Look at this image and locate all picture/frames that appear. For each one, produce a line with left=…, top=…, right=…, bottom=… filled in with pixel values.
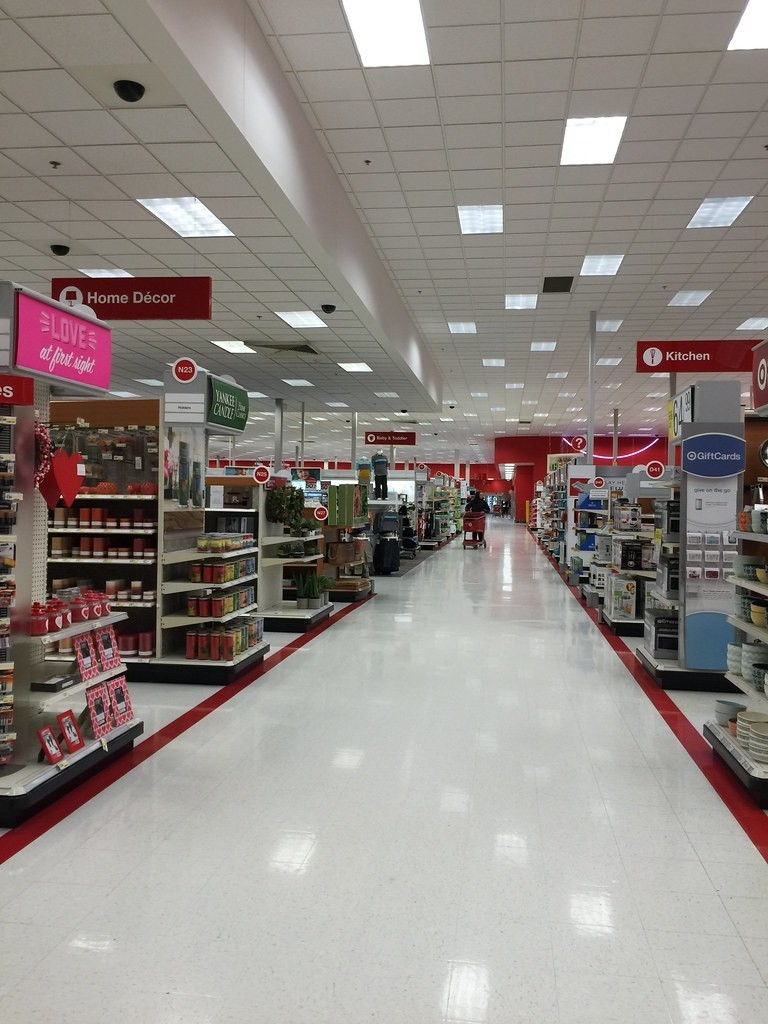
left=36, top=725, right=66, bottom=766
left=54, top=710, right=85, bottom=753
left=76, top=684, right=113, bottom=740
left=67, top=631, right=100, bottom=683
left=107, top=675, right=134, bottom=727
left=92, top=624, right=122, bottom=672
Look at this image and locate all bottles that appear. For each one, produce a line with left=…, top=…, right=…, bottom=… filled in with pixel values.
left=197, top=533, right=253, bottom=552
left=31, top=590, right=110, bottom=634
left=188, top=586, right=254, bottom=618
left=191, top=557, right=256, bottom=584
left=185, top=618, right=264, bottom=661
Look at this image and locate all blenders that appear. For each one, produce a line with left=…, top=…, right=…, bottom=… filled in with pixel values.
left=627, top=550, right=636, bottom=569
left=629, top=508, right=640, bottom=527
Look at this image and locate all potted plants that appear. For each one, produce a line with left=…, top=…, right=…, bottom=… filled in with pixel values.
left=304, top=545, right=315, bottom=556
left=314, top=575, right=328, bottom=606
left=301, top=518, right=314, bottom=538
left=315, top=521, right=322, bottom=535
left=309, top=571, right=321, bottom=609
left=314, top=545, right=321, bottom=555
left=320, top=577, right=335, bottom=604
left=292, top=572, right=309, bottom=608
left=277, top=544, right=291, bottom=558
left=291, top=543, right=305, bottom=558
left=280, top=487, right=306, bottom=537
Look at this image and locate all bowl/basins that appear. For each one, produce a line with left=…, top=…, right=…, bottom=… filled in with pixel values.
left=715, top=700, right=768, bottom=762
left=732, top=555, right=768, bottom=583
left=727, top=642, right=768, bottom=699
left=735, top=510, right=768, bottom=534
left=759, top=440, right=768, bottom=468
left=733, top=593, right=768, bottom=628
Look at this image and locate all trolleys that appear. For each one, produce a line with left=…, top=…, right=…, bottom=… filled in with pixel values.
left=492, top=505, right=501, bottom=518
left=463, top=512, right=486, bottom=550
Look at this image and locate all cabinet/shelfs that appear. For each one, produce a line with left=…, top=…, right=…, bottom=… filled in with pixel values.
left=528, top=338, right=768, bottom=812
left=0, top=281, right=477, bottom=829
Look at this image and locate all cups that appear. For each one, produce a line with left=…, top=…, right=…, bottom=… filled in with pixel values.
left=52, top=578, right=156, bottom=601
left=53, top=508, right=157, bottom=528
left=45, top=632, right=155, bottom=658
left=50, top=537, right=155, bottom=558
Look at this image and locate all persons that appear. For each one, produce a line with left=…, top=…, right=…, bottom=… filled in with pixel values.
left=464, top=491, right=490, bottom=549
left=371, top=449, right=390, bottom=500
left=296, top=469, right=316, bottom=488
left=234, top=468, right=249, bottom=475
left=354, top=457, right=373, bottom=500
left=501, top=499, right=511, bottom=520
